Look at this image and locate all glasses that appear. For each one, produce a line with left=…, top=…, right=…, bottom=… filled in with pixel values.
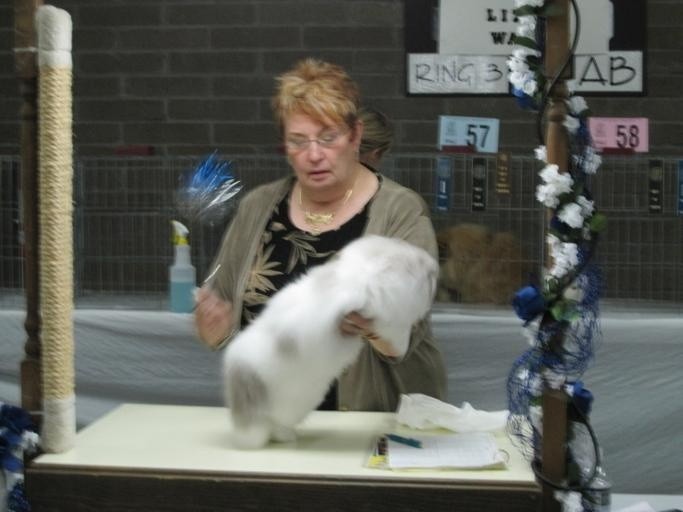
left=282, top=127, right=351, bottom=156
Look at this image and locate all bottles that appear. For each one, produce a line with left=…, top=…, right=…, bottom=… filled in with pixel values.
left=580, top=449, right=614, bottom=512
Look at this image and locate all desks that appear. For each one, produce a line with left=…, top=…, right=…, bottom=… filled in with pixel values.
left=26, top=403, right=546, bottom=512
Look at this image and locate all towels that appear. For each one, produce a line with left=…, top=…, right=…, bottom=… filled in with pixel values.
left=394, top=392, right=512, bottom=434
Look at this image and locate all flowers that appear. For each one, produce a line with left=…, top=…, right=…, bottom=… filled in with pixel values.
left=505, top=0, right=610, bottom=512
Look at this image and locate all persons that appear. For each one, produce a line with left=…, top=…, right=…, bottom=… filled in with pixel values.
left=193, top=58, right=447, bottom=410
left=354, top=107, right=400, bottom=169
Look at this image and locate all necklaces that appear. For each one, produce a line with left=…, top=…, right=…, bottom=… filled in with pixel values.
left=297, top=167, right=362, bottom=229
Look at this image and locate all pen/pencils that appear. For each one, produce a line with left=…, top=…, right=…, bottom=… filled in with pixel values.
left=385, top=435, right=421, bottom=448
